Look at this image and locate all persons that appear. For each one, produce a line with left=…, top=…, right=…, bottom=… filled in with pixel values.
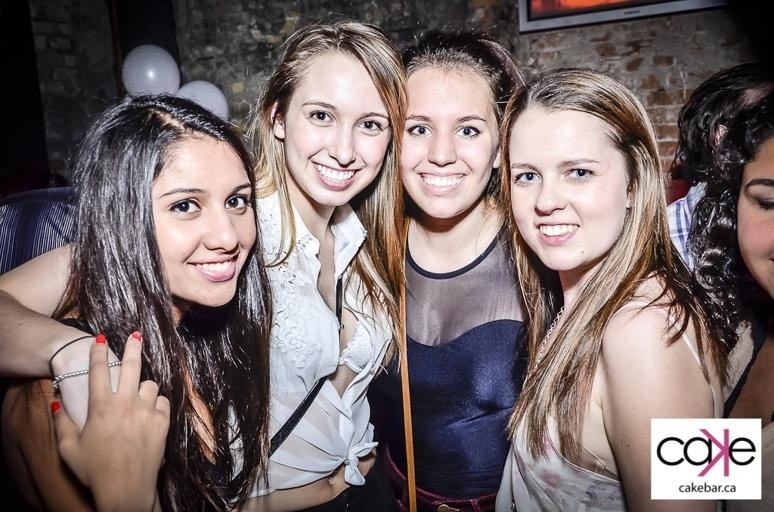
left=0, top=92, right=272, bottom=512
left=1, top=22, right=409, bottom=511
left=658, top=64, right=773, bottom=510
left=363, top=29, right=565, bottom=512
left=690, top=95, right=773, bottom=512
left=492, top=63, right=733, bottom=511
left=668, top=60, right=774, bottom=271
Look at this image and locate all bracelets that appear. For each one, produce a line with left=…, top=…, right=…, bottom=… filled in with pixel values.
left=48, top=358, right=121, bottom=389
left=48, top=334, right=94, bottom=361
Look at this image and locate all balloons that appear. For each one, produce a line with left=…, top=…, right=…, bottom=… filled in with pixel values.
left=121, top=43, right=180, bottom=99
left=178, top=80, right=231, bottom=124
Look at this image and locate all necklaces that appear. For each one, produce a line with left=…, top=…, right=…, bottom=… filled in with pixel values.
left=533, top=305, right=565, bottom=362
